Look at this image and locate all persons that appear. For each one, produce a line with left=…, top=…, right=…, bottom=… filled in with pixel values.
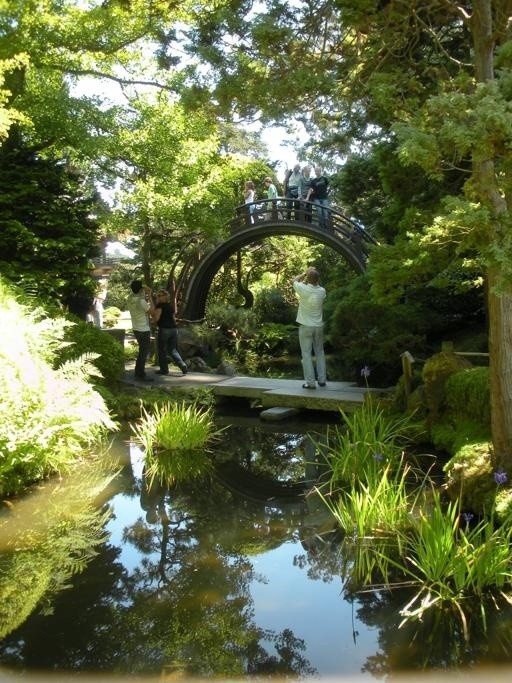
left=242, top=180, right=262, bottom=225
left=283, top=162, right=302, bottom=221
left=296, top=427, right=346, bottom=570
left=139, top=454, right=168, bottom=525
left=302, top=165, right=330, bottom=229
left=292, top=265, right=328, bottom=389
left=126, top=279, right=155, bottom=381
left=90, top=279, right=108, bottom=328
left=297, top=162, right=312, bottom=224
left=148, top=288, right=189, bottom=375
left=261, top=175, right=284, bottom=222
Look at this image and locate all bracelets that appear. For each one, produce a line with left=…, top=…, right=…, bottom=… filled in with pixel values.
left=303, top=271, right=306, bottom=276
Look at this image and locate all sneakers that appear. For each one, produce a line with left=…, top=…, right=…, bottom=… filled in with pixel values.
left=304, top=384, right=316, bottom=389
left=318, top=383, right=326, bottom=386
left=135, top=376, right=154, bottom=381
left=155, top=370, right=168, bottom=374
left=182, top=365, right=188, bottom=373
